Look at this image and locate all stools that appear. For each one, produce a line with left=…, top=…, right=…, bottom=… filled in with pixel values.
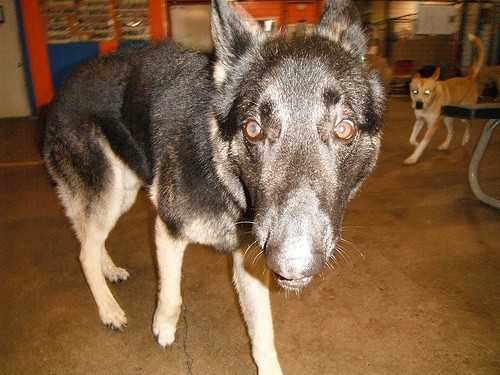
left=440, top=102, right=500, bottom=211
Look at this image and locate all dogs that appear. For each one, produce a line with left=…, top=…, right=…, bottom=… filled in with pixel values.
left=401, top=34, right=485, bottom=164
left=41, top=0, right=388, bottom=375
